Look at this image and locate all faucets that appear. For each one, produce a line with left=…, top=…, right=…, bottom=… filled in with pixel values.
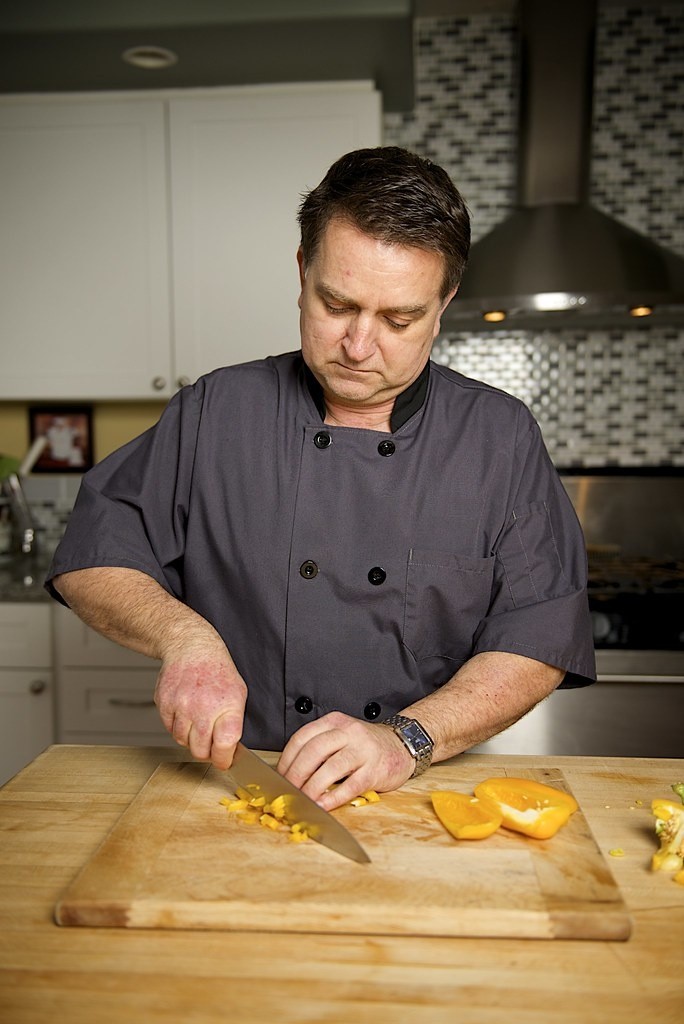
left=3, top=471, right=40, bottom=587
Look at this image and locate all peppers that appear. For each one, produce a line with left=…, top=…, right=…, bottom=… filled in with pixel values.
left=219, top=775, right=683, bottom=886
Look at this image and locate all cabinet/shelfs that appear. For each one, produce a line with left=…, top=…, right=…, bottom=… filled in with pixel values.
left=1, top=600, right=178, bottom=790
left=0, top=78, right=382, bottom=405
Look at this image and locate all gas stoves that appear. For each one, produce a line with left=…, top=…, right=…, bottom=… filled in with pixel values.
left=577, top=548, right=681, bottom=650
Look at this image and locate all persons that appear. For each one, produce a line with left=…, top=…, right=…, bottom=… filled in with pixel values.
left=37, top=143, right=596, bottom=817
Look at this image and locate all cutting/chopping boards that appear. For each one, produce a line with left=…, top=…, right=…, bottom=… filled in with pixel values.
left=55, top=753, right=630, bottom=942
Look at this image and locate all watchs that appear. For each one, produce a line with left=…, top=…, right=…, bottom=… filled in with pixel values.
left=380, top=716, right=435, bottom=779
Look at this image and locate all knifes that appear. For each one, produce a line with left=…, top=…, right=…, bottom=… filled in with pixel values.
left=223, top=744, right=371, bottom=867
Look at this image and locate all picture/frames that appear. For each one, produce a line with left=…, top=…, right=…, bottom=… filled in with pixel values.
left=25, top=400, right=96, bottom=476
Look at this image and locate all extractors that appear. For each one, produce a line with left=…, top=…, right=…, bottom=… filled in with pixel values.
left=421, top=0, right=684, bottom=332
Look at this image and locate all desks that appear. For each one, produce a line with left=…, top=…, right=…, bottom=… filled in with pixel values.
left=0, top=742, right=684, bottom=1024
